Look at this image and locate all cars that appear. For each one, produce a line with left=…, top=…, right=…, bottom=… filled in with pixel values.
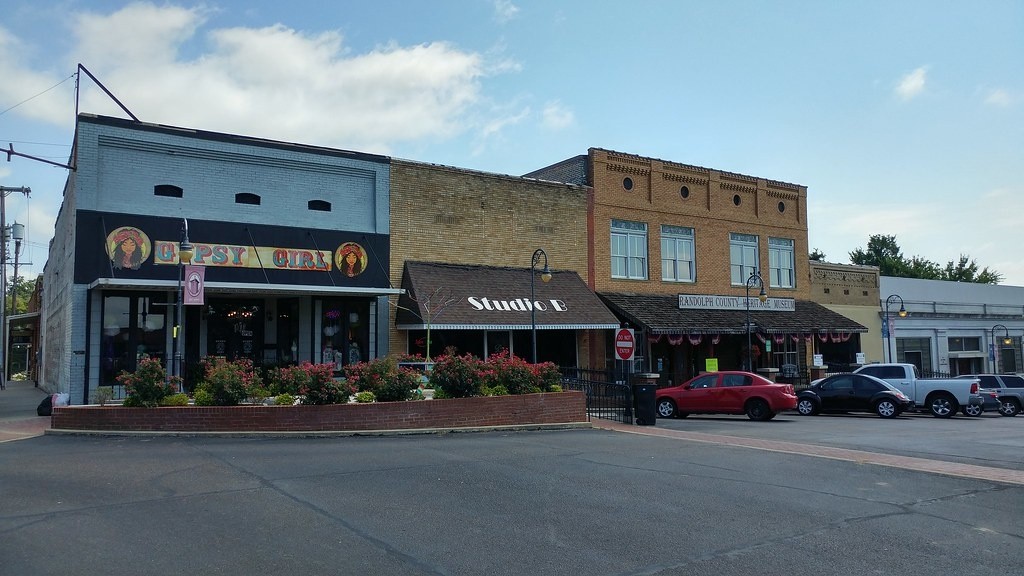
left=962, top=388, right=1002, bottom=417
left=952, top=372, right=1024, bottom=417
left=656, top=371, right=798, bottom=421
left=103, top=345, right=165, bottom=375
left=795, top=374, right=915, bottom=419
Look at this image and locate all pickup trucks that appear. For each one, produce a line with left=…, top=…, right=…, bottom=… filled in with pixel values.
left=811, top=362, right=984, bottom=419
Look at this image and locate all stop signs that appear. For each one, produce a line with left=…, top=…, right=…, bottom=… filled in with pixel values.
left=615, top=328, right=635, bottom=361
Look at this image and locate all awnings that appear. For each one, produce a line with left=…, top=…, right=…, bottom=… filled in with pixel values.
left=595, top=291, right=869, bottom=333
left=89, top=216, right=406, bottom=296
left=395, top=261, right=620, bottom=330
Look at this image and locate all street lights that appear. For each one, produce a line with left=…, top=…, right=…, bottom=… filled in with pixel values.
left=886, top=294, right=907, bottom=362
left=173, top=217, right=194, bottom=393
left=531, top=249, right=552, bottom=363
left=992, top=325, right=1012, bottom=373
left=747, top=274, right=767, bottom=371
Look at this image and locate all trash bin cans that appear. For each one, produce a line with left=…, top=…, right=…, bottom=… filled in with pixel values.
left=632, top=384, right=657, bottom=425
